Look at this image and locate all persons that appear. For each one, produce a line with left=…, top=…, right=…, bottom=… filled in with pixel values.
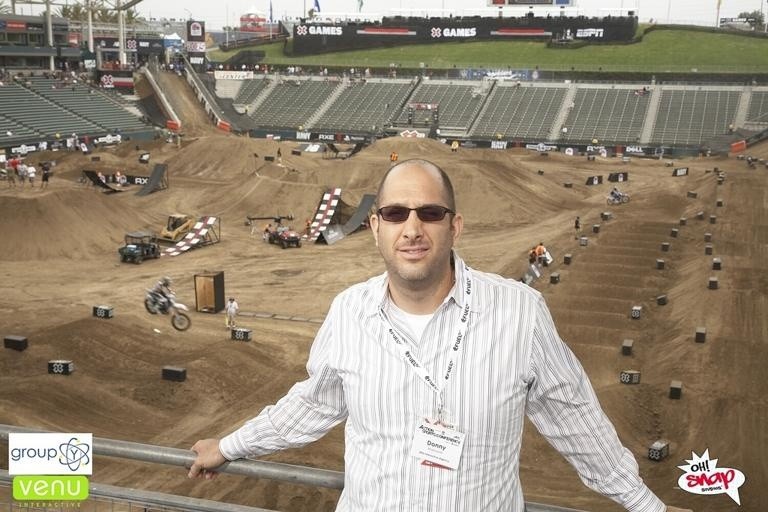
left=152, top=276, right=175, bottom=315
left=536, top=243, right=546, bottom=267
left=528, top=247, right=537, bottom=266
left=263, top=224, right=272, bottom=240
left=186, top=159, right=695, bottom=512
left=390, top=151, right=398, bottom=166
left=1, top=69, right=121, bottom=190
left=305, top=218, right=312, bottom=239
left=574, top=216, right=581, bottom=240
left=611, top=187, right=620, bottom=202
left=225, top=297, right=239, bottom=328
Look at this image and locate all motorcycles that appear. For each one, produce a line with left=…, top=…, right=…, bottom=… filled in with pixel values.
left=143, top=287, right=191, bottom=331
left=606, top=192, right=630, bottom=206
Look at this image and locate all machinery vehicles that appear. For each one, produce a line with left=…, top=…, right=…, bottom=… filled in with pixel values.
left=149, top=213, right=196, bottom=248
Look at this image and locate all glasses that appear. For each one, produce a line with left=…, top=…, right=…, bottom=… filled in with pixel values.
left=377, top=205, right=455, bottom=221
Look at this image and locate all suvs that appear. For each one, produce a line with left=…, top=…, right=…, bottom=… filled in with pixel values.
left=269, top=225, right=301, bottom=248
left=118, top=231, right=160, bottom=264
